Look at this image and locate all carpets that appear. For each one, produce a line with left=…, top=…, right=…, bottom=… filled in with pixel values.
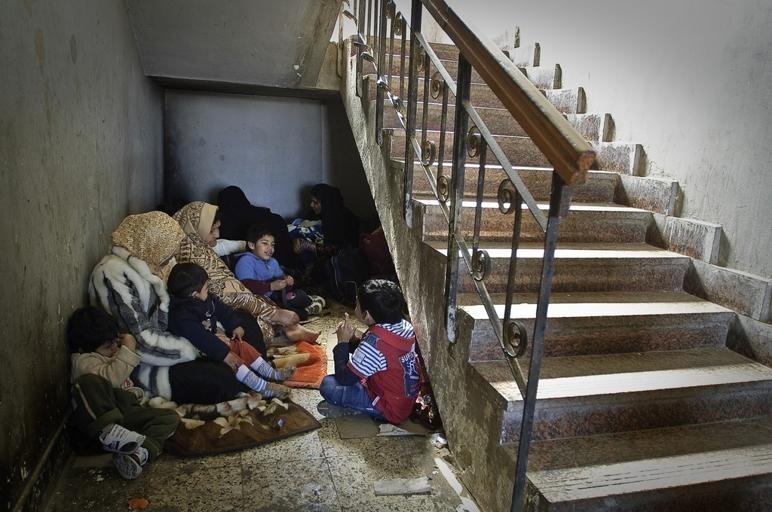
left=279, top=342, right=327, bottom=387
left=161, top=396, right=320, bottom=458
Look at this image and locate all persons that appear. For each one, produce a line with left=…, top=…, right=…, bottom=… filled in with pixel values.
left=88, top=186, right=326, bottom=400
left=61, top=306, right=179, bottom=480
left=290, top=184, right=361, bottom=299
left=319, top=278, right=422, bottom=425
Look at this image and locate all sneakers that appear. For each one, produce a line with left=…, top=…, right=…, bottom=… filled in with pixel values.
left=269, top=367, right=295, bottom=382
left=113, top=447, right=149, bottom=480
left=265, top=383, right=291, bottom=399
left=99, top=425, right=146, bottom=456
left=306, top=294, right=326, bottom=315
